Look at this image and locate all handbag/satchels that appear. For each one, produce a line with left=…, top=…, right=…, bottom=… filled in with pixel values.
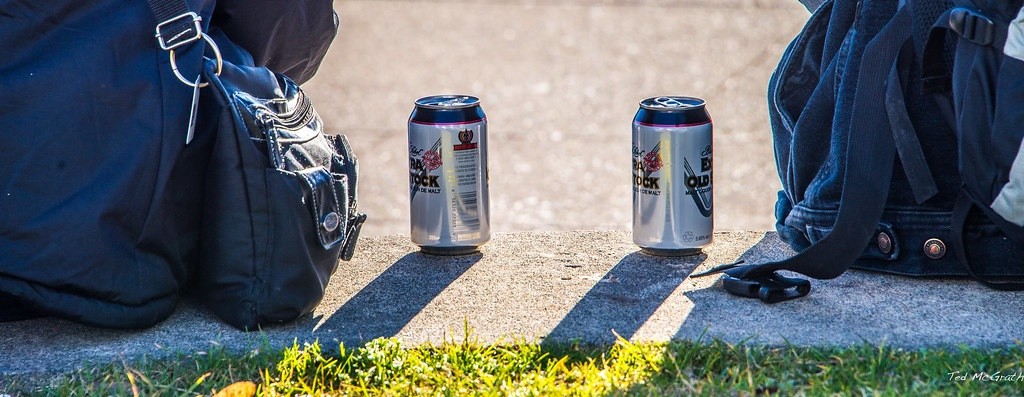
left=200, top=20, right=368, bottom=332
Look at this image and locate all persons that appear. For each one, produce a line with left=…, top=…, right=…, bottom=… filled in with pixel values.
left=769, top=0, right=1024, bottom=285
left=0, top=0, right=338, bottom=329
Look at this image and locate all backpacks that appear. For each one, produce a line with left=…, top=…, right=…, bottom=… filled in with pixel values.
left=722, top=0, right=1023, bottom=305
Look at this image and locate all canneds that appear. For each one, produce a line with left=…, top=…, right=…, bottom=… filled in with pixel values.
left=632, top=96, right=713, bottom=256
left=407, top=95, right=489, bottom=254
